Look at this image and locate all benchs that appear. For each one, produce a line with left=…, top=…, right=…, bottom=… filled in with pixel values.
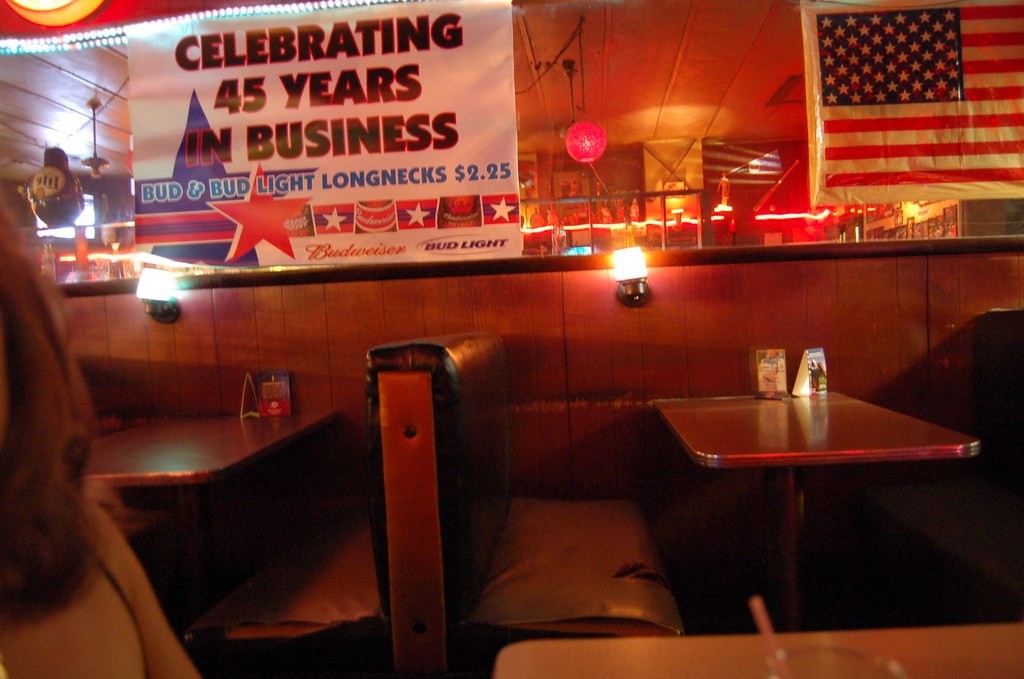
left=76, top=292, right=1024, bottom=677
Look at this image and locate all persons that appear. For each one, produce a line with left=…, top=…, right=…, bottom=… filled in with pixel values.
left=0, top=250, right=204, bottom=679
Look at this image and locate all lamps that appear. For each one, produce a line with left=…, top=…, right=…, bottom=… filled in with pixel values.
left=610, top=244, right=655, bottom=306
left=137, top=266, right=184, bottom=323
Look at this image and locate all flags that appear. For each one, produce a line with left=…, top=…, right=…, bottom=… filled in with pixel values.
left=799, top=0, right=1024, bottom=206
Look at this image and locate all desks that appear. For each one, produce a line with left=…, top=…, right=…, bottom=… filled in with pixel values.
left=657, top=392, right=985, bottom=627
left=81, top=403, right=345, bottom=619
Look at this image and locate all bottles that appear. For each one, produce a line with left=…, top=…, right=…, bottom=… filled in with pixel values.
left=42, top=243, right=57, bottom=281
